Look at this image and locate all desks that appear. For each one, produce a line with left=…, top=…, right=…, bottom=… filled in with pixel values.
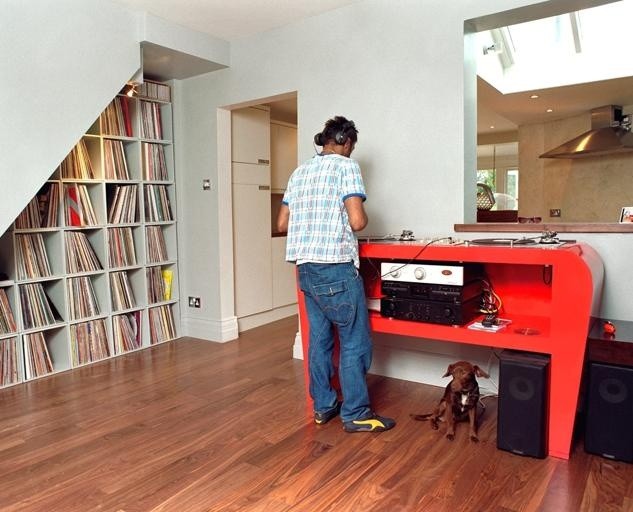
left=295, top=240, right=605, bottom=460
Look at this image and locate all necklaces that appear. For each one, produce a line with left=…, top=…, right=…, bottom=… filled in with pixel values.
left=321, top=148, right=336, bottom=154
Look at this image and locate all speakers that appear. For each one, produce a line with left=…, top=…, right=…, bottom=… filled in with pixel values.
left=496, top=348, right=550, bottom=460
left=584, top=362, right=633, bottom=463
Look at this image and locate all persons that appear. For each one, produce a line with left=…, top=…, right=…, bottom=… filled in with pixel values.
left=276, top=114, right=396, bottom=434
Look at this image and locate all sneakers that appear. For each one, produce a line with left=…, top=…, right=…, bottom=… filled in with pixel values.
left=342, top=415, right=396, bottom=434
left=313, top=400, right=341, bottom=425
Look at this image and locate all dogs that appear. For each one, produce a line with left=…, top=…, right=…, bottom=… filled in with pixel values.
left=408, top=360, right=491, bottom=443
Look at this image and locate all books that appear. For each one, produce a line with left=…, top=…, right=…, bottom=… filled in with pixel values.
left=100, top=81, right=178, bottom=355
left=0, top=280, right=18, bottom=385
left=15, top=183, right=99, bottom=229
left=61, top=138, right=95, bottom=179
left=17, top=231, right=101, bottom=382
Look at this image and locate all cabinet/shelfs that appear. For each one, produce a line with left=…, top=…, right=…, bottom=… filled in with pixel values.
left=1, top=80, right=181, bottom=390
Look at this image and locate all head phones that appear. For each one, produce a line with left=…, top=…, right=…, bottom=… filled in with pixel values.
left=313, top=120, right=355, bottom=145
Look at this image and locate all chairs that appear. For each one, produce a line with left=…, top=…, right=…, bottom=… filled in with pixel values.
left=231, top=104, right=298, bottom=333
